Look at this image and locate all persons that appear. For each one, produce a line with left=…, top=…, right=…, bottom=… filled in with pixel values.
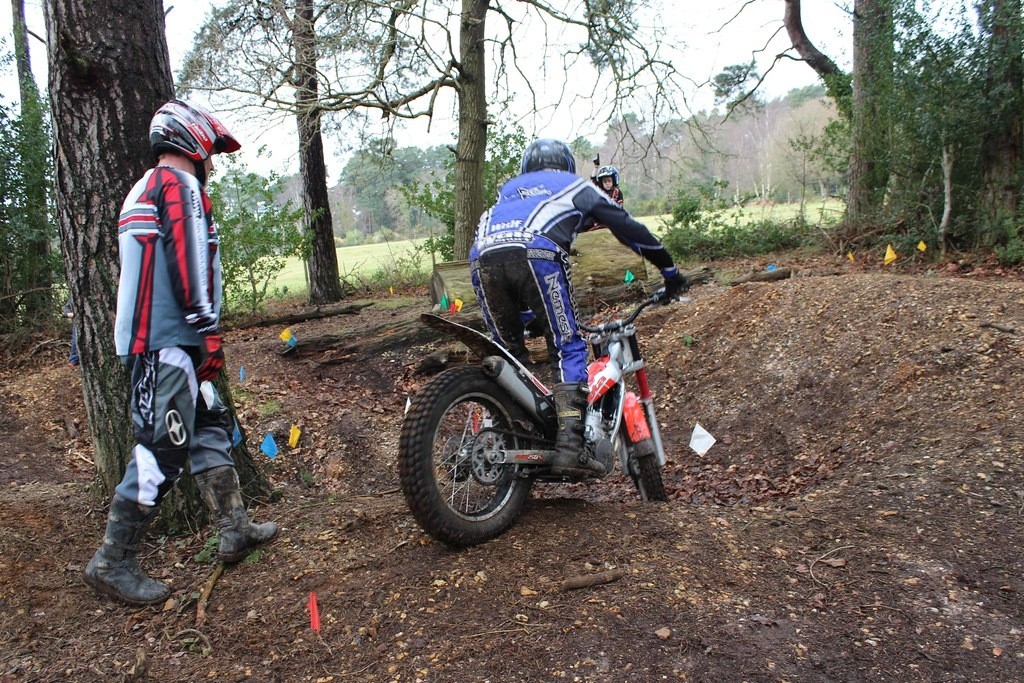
left=587, top=153, right=623, bottom=231
left=65, top=298, right=80, bottom=367
left=82, top=98, right=282, bottom=605
left=469, top=139, right=686, bottom=479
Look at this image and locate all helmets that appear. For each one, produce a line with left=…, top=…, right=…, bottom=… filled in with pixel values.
left=596, top=166, right=619, bottom=189
left=149, top=99, right=241, bottom=161
left=521, top=138, right=576, bottom=174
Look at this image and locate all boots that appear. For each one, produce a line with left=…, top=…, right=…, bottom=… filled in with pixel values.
left=82, top=493, right=170, bottom=606
left=552, top=381, right=605, bottom=478
left=195, top=465, right=280, bottom=562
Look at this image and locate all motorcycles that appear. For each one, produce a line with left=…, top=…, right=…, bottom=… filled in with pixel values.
left=393, top=278, right=694, bottom=547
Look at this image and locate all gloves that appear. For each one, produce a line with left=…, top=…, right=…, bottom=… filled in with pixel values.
left=663, top=271, right=690, bottom=307
left=525, top=317, right=545, bottom=339
left=593, top=153, right=599, bottom=168
left=195, top=329, right=225, bottom=385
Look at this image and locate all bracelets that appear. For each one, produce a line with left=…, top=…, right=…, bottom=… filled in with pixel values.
left=196, top=324, right=218, bottom=335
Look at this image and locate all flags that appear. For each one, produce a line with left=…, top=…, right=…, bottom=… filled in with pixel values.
left=280, top=327, right=297, bottom=348
left=849, top=251, right=855, bottom=267
left=766, top=263, right=778, bottom=271
left=624, top=269, right=635, bottom=285
left=441, top=286, right=463, bottom=315
left=306, top=591, right=320, bottom=635
left=289, top=424, right=301, bottom=449
left=884, top=243, right=897, bottom=266
left=239, top=365, right=245, bottom=383
left=390, top=286, right=393, bottom=296
left=260, top=432, right=278, bottom=459
left=690, top=424, right=716, bottom=458
left=917, top=240, right=927, bottom=251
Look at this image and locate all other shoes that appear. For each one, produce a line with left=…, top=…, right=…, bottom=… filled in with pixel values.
left=67, top=362, right=80, bottom=367
